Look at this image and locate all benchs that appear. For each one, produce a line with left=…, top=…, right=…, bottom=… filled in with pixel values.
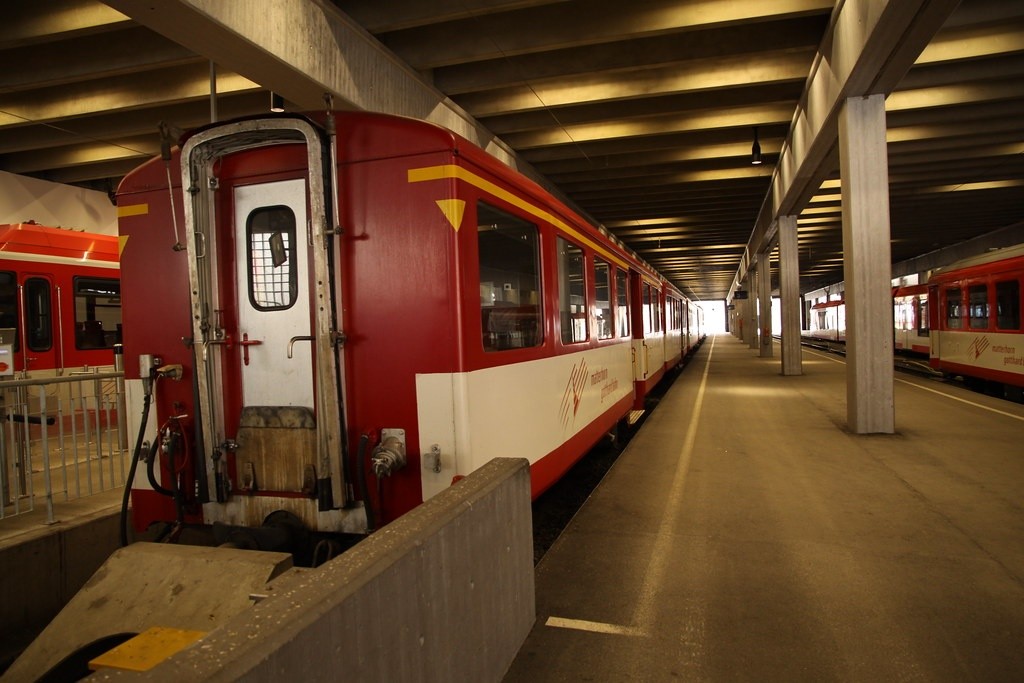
left=488, top=305, right=541, bottom=346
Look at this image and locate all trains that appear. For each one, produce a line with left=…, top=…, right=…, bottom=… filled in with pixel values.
left=0, top=221, right=123, bottom=442
left=117, top=110, right=706, bottom=569
left=744, top=244, right=1024, bottom=402
